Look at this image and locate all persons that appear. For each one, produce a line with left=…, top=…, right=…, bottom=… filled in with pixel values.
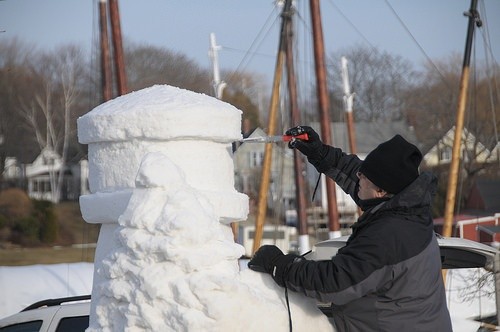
left=248, top=125, right=453, bottom=331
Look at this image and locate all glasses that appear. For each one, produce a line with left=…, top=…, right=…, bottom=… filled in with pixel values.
left=355, top=172, right=377, bottom=188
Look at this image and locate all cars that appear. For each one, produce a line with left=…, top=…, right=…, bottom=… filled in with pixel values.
left=235, top=220, right=352, bottom=261
left=435, top=231, right=499, bottom=323
left=0, top=294, right=93, bottom=332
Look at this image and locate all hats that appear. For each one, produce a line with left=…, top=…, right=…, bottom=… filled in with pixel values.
left=360, top=134, right=423, bottom=195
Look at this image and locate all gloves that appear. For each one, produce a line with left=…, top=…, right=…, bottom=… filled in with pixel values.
left=247, top=244, right=295, bottom=286
left=286, top=125, right=335, bottom=172
